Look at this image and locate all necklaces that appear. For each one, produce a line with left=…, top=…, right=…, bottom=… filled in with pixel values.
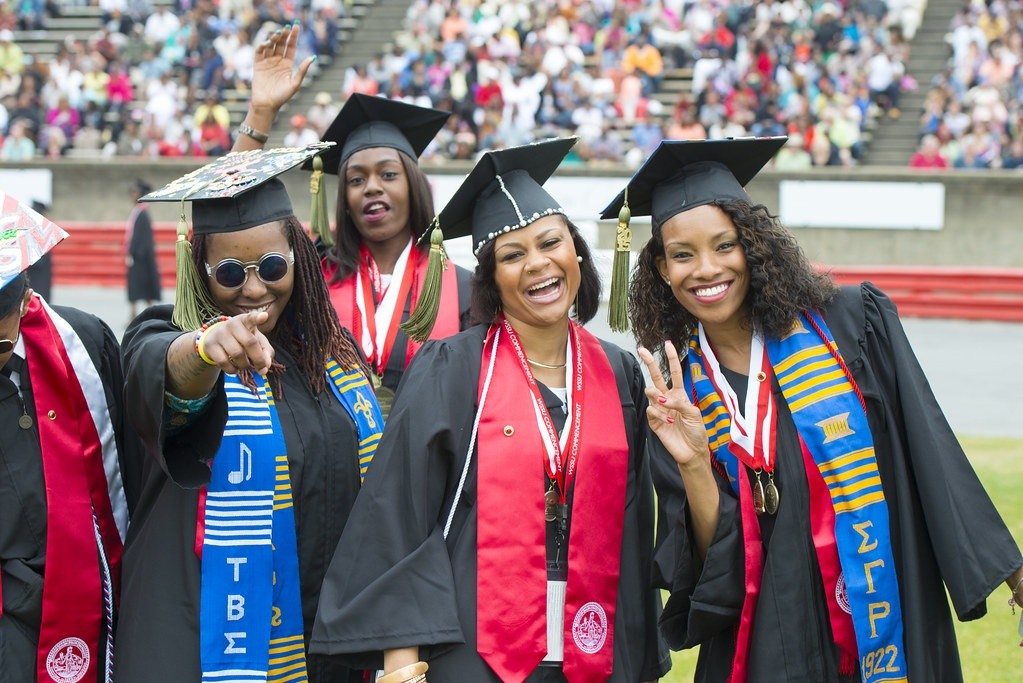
left=527, top=359, right=570, bottom=368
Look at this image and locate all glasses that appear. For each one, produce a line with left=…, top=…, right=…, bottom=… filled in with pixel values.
left=202, top=247, right=295, bottom=291
left=0, top=299, right=25, bottom=354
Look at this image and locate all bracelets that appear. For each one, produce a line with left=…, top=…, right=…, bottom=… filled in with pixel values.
left=1008, top=577, right=1023, bottom=615
left=237, top=123, right=269, bottom=144
left=375, top=661, right=430, bottom=683
left=193, top=315, right=232, bottom=367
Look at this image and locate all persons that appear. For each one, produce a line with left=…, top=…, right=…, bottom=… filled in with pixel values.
left=599, top=133, right=1023, bottom=683
left=230, top=19, right=474, bottom=389
left=0, top=1, right=1023, bottom=168
left=308, top=135, right=677, bottom=683
left=115, top=141, right=385, bottom=683
left=0, top=190, right=142, bottom=683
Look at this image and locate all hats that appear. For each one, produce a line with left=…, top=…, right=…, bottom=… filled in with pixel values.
left=0, top=189, right=70, bottom=303
left=300, top=92, right=451, bottom=248
left=398, top=136, right=581, bottom=344
left=599, top=135, right=790, bottom=333
left=136, top=141, right=336, bottom=332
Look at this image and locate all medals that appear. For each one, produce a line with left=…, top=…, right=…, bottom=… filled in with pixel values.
left=753, top=479, right=779, bottom=515
left=544, top=491, right=559, bottom=521
